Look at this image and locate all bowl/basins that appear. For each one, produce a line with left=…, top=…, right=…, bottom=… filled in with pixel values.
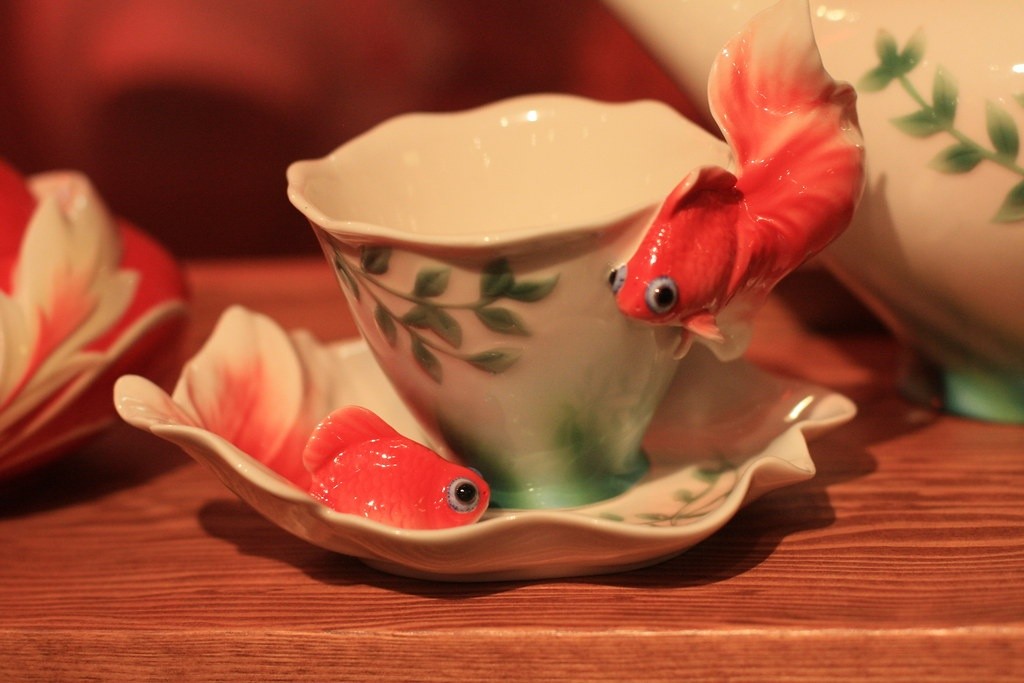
left=597, top=0, right=1024, bottom=423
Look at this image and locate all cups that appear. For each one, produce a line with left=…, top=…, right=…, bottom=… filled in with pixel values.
left=287, top=92, right=743, bottom=510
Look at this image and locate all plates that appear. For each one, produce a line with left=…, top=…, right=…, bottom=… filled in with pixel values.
left=112, top=305, right=858, bottom=580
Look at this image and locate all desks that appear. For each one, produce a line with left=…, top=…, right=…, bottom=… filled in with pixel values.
left=0, top=253, right=1024, bottom=683
